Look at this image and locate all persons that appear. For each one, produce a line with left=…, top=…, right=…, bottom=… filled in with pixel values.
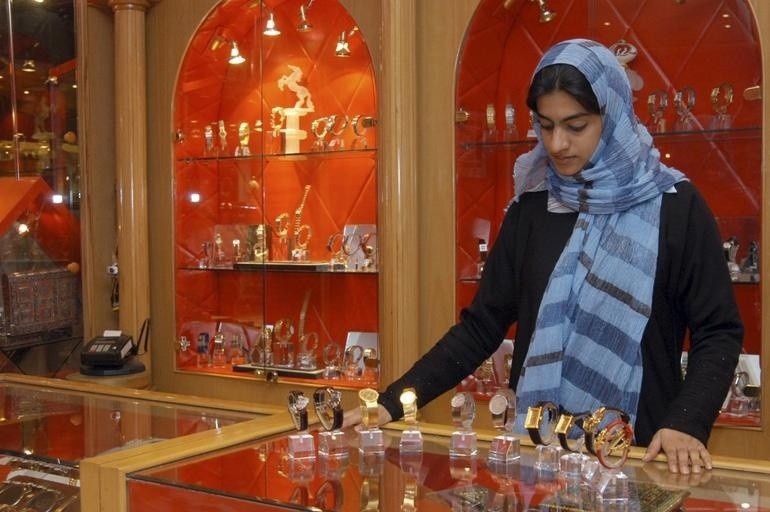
left=317, top=39, right=743, bottom=474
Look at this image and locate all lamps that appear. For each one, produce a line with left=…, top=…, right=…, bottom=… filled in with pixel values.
left=248, top=0, right=316, bottom=37
left=210, top=30, right=247, bottom=66
left=536, top=0, right=558, bottom=23
left=333, top=25, right=359, bottom=58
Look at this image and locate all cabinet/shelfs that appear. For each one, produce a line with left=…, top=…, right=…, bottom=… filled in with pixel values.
left=461, top=124, right=763, bottom=433
left=176, top=146, right=381, bottom=393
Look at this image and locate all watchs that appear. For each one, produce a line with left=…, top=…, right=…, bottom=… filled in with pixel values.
left=202, top=213, right=361, bottom=255
left=204, top=120, right=251, bottom=148
left=582, top=406, right=630, bottom=457
left=287, top=390, right=310, bottom=431
left=310, top=112, right=369, bottom=137
left=485, top=105, right=515, bottom=128
left=489, top=389, right=516, bottom=432
left=478, top=238, right=488, bottom=260
left=313, top=385, right=343, bottom=431
left=649, top=86, right=734, bottom=113
left=197, top=319, right=376, bottom=369
left=554, top=411, right=581, bottom=453
left=595, top=420, right=633, bottom=469
left=525, top=400, right=559, bottom=446
left=482, top=354, right=513, bottom=381
left=449, top=392, right=476, bottom=427
left=358, top=388, right=380, bottom=428
left=399, top=387, right=419, bottom=424
left=723, top=237, right=739, bottom=261
left=731, top=370, right=749, bottom=400
